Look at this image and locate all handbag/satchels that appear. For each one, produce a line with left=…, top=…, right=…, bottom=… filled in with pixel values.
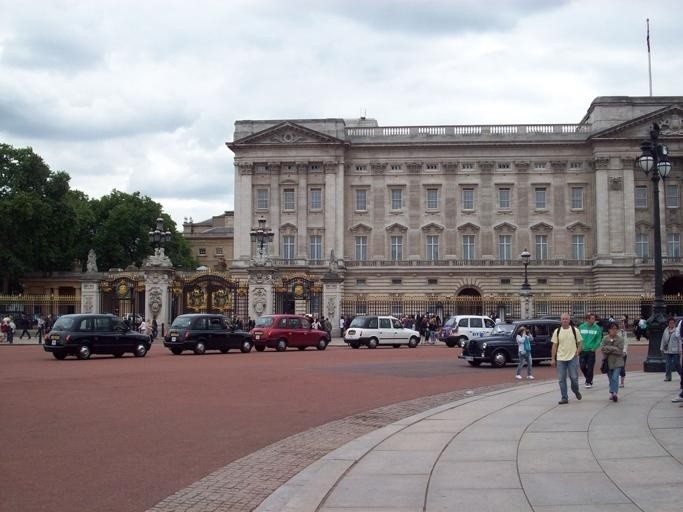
left=601, top=360, right=608, bottom=373
left=664, top=344, right=668, bottom=350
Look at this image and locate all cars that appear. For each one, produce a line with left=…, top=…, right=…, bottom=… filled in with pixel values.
left=456, top=320, right=560, bottom=368
left=247, top=314, right=329, bottom=351
left=161, top=313, right=253, bottom=354
left=121, top=312, right=143, bottom=323
left=40, top=313, right=151, bottom=359
left=342, top=315, right=420, bottom=348
left=538, top=315, right=581, bottom=329
left=673, top=316, right=683, bottom=322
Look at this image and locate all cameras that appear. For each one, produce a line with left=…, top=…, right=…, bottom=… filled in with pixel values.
left=526, top=330, right=529, bottom=334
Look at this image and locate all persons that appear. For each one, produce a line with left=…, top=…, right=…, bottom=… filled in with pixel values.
left=401, top=312, right=442, bottom=344
left=19, top=312, right=32, bottom=341
left=576, top=312, right=682, bottom=407
left=551, top=313, right=582, bottom=405
left=515, top=327, right=534, bottom=379
left=233, top=316, right=256, bottom=333
left=123, top=314, right=158, bottom=344
left=34, top=313, right=59, bottom=337
left=308, top=315, right=332, bottom=343
left=490, top=313, right=498, bottom=322
left=1, top=314, right=16, bottom=344
left=339, top=316, right=354, bottom=337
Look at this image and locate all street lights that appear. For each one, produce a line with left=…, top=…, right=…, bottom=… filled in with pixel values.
left=519, top=247, right=531, bottom=289
left=633, top=121, right=676, bottom=372
left=250, top=215, right=274, bottom=257
left=147, top=213, right=171, bottom=249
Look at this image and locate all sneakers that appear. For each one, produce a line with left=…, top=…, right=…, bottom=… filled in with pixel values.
left=514, top=374, right=535, bottom=380
left=671, top=396, right=683, bottom=402
left=575, top=392, right=582, bottom=400
left=559, top=400, right=568, bottom=404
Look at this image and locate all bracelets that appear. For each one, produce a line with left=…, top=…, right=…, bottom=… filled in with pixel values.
left=576, top=351, right=578, bottom=355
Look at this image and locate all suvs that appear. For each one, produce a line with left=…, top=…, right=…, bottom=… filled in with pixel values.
left=0, top=310, right=38, bottom=329
left=434, top=314, right=513, bottom=348
